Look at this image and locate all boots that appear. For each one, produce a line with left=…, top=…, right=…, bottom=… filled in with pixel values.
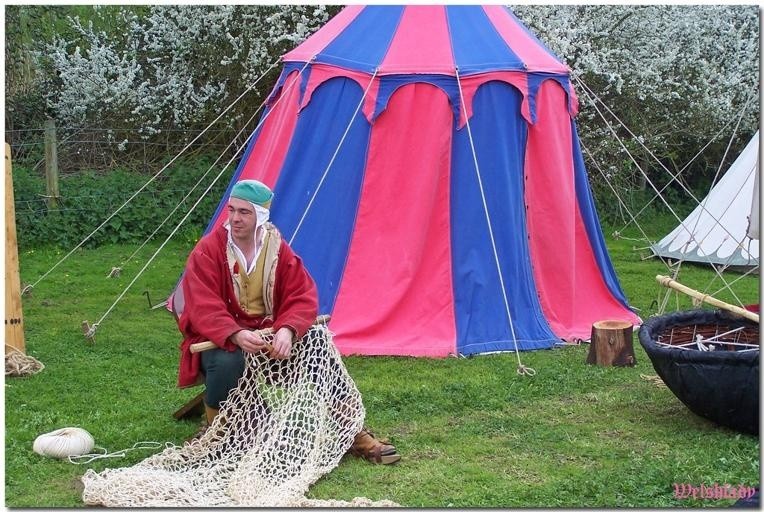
left=333, top=397, right=395, bottom=457
left=185, top=404, right=232, bottom=454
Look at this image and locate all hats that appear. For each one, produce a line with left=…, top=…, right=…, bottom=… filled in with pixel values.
left=230, top=180, right=273, bottom=210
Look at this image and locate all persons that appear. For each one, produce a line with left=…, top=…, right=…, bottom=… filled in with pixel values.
left=171, top=178, right=400, bottom=465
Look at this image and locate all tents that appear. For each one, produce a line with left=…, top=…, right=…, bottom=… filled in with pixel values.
left=651, top=129, right=762, bottom=266
left=168, top=5, right=642, bottom=358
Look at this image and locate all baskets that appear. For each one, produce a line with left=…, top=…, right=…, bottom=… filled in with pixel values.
left=640, top=309, right=759, bottom=435
